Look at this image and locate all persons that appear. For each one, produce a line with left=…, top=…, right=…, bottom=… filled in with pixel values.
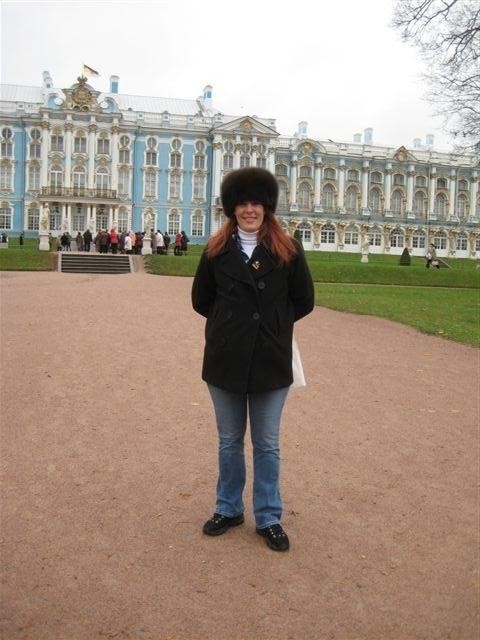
left=96, top=228, right=171, bottom=254
left=49, top=233, right=52, bottom=248
left=426, top=243, right=440, bottom=269
left=20, top=234, right=24, bottom=245
left=174, top=231, right=190, bottom=256
left=60, top=232, right=73, bottom=251
left=76, top=229, right=92, bottom=251
left=192, top=168, right=315, bottom=551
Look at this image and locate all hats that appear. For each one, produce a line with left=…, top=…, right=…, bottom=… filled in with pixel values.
left=221, top=167, right=279, bottom=218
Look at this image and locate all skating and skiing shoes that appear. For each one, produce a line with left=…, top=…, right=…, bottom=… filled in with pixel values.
left=256, top=524, right=289, bottom=551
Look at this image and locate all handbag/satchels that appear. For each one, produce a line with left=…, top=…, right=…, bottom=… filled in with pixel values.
left=291, top=336, right=306, bottom=389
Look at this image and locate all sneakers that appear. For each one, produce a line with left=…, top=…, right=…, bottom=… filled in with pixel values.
left=203, top=513, right=244, bottom=536
